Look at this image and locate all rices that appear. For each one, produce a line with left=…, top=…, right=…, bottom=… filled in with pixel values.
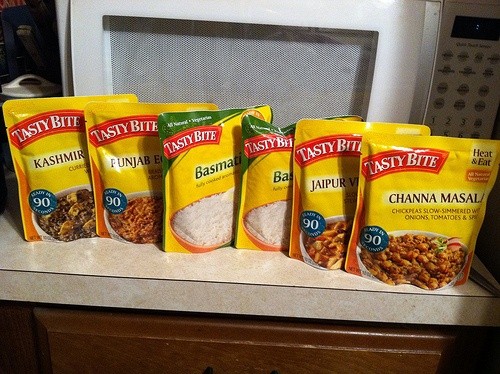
left=171, top=192, right=292, bottom=247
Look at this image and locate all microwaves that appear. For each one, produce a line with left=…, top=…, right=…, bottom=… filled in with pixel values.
left=53, top=0, right=500, bottom=138
